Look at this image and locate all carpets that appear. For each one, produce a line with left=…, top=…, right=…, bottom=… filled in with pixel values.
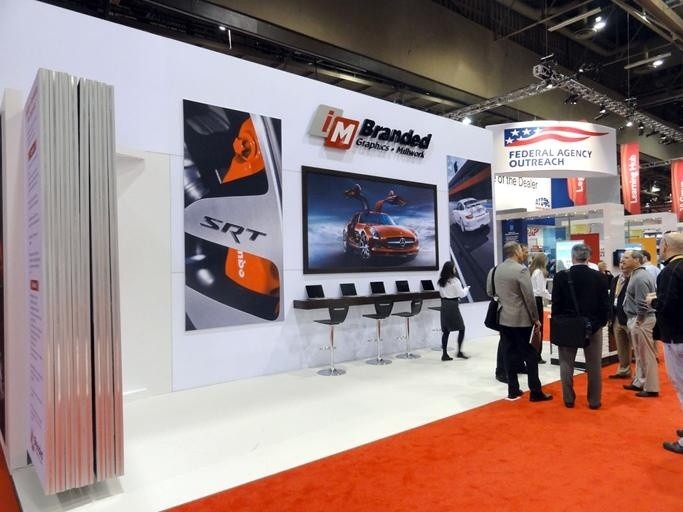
left=163, top=336, right=681, bottom=511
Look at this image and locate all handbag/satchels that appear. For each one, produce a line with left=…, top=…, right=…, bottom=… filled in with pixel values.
left=484, top=300, right=499, bottom=331
left=550, top=314, right=592, bottom=348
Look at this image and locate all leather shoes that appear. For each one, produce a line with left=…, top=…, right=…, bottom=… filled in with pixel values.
left=663, top=441, right=683, bottom=453
left=442, top=353, right=467, bottom=360
left=496, top=359, right=601, bottom=408
left=609, top=375, right=658, bottom=397
left=677, top=429, right=683, bottom=436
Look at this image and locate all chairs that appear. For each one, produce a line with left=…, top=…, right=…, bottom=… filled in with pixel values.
left=362, top=297, right=393, bottom=366
left=391, top=298, right=423, bottom=360
left=313, top=300, right=350, bottom=377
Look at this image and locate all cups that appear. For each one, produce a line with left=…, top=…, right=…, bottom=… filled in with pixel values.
left=646, top=292, right=657, bottom=313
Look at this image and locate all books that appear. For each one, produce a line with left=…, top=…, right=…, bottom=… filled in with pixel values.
left=529, top=323, right=542, bottom=353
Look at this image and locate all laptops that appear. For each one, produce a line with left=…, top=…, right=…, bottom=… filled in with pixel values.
left=370, top=282, right=397, bottom=297
left=305, top=285, right=339, bottom=300
left=340, top=283, right=370, bottom=298
left=421, top=280, right=439, bottom=292
left=395, top=280, right=419, bottom=294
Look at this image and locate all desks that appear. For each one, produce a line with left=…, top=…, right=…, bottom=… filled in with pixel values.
left=545, top=277, right=554, bottom=294
left=548, top=314, right=619, bottom=372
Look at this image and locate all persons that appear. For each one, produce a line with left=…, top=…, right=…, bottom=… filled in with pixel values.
left=644, top=231, right=683, bottom=454
left=551, top=243, right=608, bottom=410
left=437, top=261, right=471, bottom=361
left=597, top=248, right=664, bottom=397
left=495, top=243, right=547, bottom=383
left=486, top=241, right=553, bottom=402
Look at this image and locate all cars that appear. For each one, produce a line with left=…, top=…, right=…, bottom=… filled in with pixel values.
left=449, top=196, right=490, bottom=236
left=339, top=183, right=421, bottom=261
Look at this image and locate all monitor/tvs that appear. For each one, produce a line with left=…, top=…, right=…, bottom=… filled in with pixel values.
left=613, top=249, right=626, bottom=266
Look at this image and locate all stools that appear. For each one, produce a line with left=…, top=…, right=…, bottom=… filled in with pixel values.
left=428, top=306, right=455, bottom=351
